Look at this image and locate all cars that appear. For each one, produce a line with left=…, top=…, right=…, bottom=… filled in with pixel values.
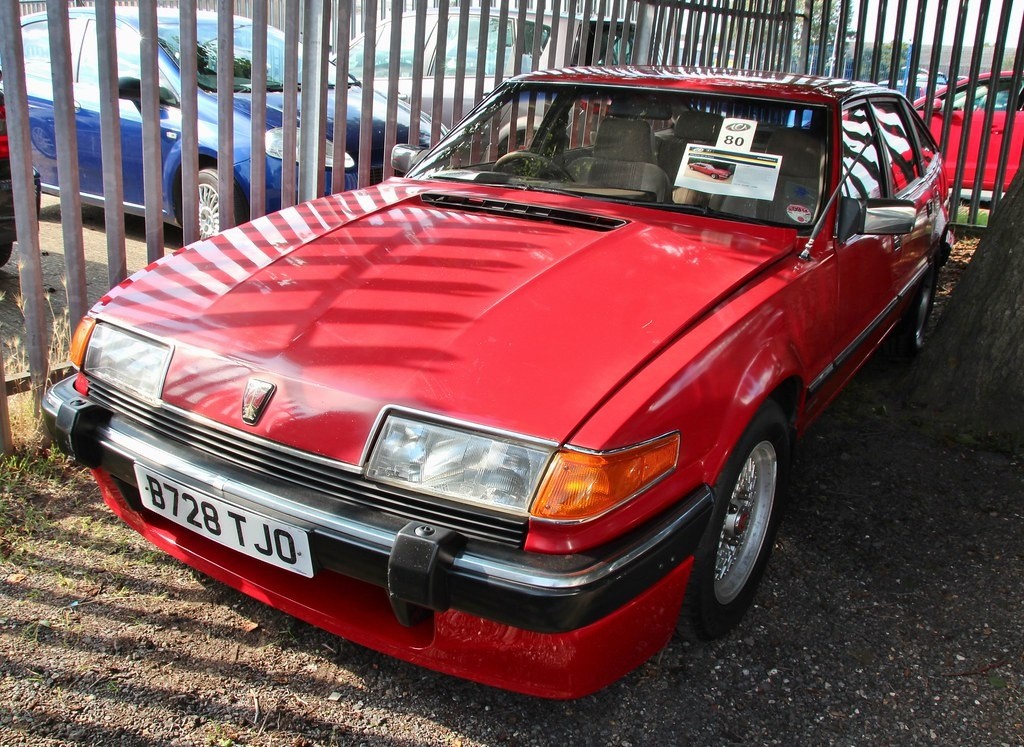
left=0, top=6, right=453, bottom=238
left=695, top=66, right=969, bottom=126
left=45, top=65, right=954, bottom=702
left=843, top=69, right=1024, bottom=192
left=328, top=5, right=672, bottom=162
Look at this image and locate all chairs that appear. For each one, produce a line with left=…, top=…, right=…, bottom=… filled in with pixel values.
left=719, top=127, right=826, bottom=226
left=750, top=122, right=776, bottom=153
left=656, top=110, right=724, bottom=208
left=564, top=117, right=672, bottom=203
